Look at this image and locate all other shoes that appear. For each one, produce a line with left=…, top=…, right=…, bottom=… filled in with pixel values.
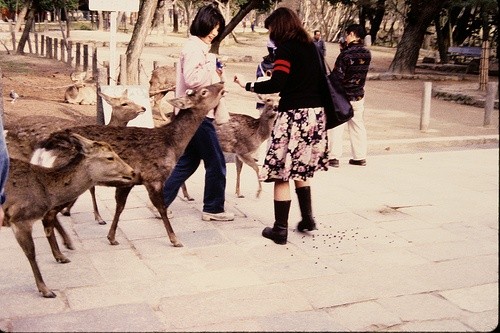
left=348, top=160, right=370, bottom=165
left=201, top=207, right=235, bottom=223
left=144, top=202, right=175, bottom=220
left=328, top=157, right=341, bottom=170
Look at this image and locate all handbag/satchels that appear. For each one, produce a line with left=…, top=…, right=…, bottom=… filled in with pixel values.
left=312, top=43, right=355, bottom=131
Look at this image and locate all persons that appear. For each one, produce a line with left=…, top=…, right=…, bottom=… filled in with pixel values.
left=147, top=4, right=235, bottom=221
left=327, top=23, right=372, bottom=167
left=233, top=7, right=330, bottom=245
left=250, top=16, right=256, bottom=32
left=312, top=30, right=327, bottom=62
left=242, top=17, right=247, bottom=32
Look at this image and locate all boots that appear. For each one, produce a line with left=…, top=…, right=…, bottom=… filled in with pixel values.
left=262, top=199, right=294, bottom=246
left=293, top=185, right=318, bottom=233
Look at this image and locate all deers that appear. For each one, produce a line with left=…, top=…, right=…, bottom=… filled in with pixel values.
left=0, top=133, right=136, bottom=298
left=4, top=89, right=147, bottom=225
left=31, top=81, right=226, bottom=247
left=149, top=66, right=176, bottom=121
left=182, top=94, right=281, bottom=201
left=65, top=72, right=96, bottom=104
